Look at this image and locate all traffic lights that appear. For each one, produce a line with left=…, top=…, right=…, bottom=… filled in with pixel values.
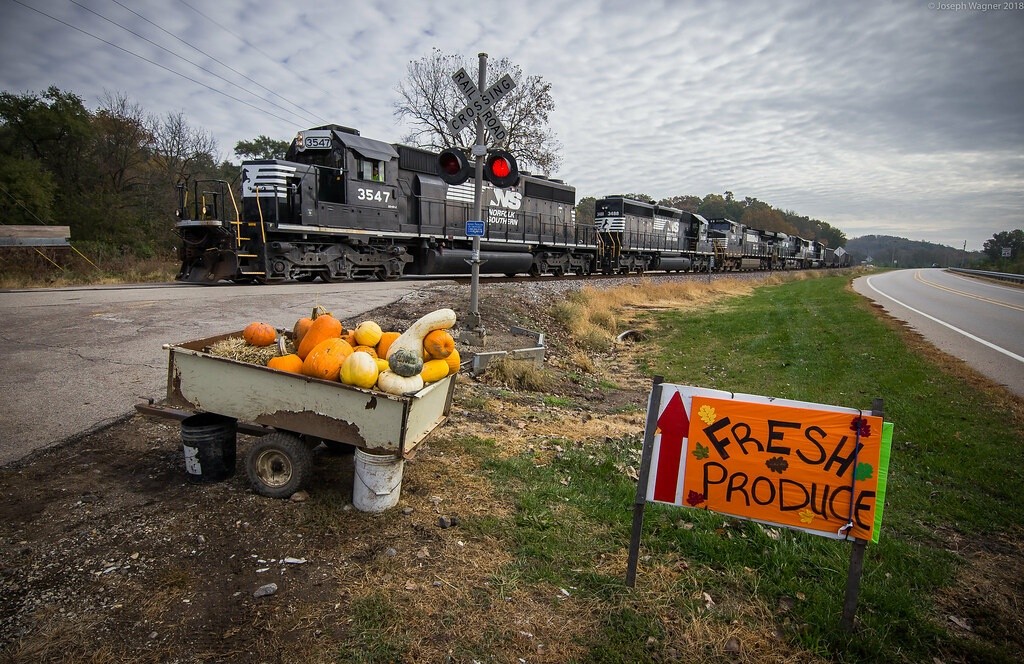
left=484, top=149, right=520, bottom=188
left=435, top=148, right=470, bottom=186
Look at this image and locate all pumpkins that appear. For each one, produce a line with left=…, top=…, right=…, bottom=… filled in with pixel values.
left=242, top=306, right=460, bottom=394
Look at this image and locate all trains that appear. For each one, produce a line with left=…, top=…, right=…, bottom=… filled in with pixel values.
left=174, top=124, right=854, bottom=287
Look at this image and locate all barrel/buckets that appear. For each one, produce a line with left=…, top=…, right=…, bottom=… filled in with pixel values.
left=180, top=413, right=240, bottom=485
left=352, top=445, right=404, bottom=512
